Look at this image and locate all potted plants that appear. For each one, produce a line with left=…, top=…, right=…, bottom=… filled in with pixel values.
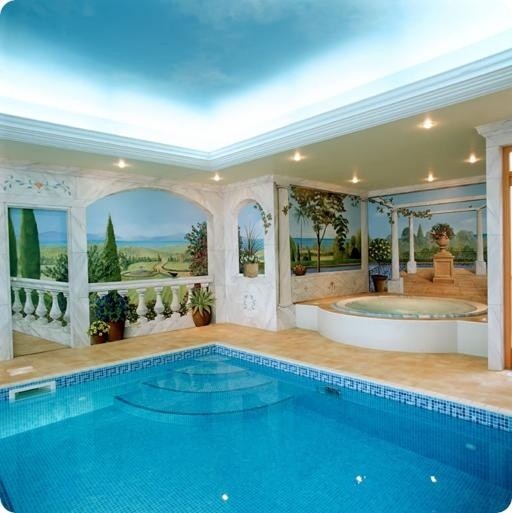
left=368, top=238, right=392, bottom=292
left=189, top=286, right=217, bottom=326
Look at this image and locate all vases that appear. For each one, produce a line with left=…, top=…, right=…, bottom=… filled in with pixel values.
left=93, top=334, right=108, bottom=343
left=436, top=237, right=451, bottom=254
left=243, top=263, right=258, bottom=277
left=107, top=321, right=125, bottom=341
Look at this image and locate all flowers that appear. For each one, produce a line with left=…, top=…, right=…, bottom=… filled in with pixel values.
left=95, top=290, right=133, bottom=322
left=430, top=222, right=455, bottom=240
left=240, top=220, right=264, bottom=263
left=87, top=320, right=111, bottom=335
